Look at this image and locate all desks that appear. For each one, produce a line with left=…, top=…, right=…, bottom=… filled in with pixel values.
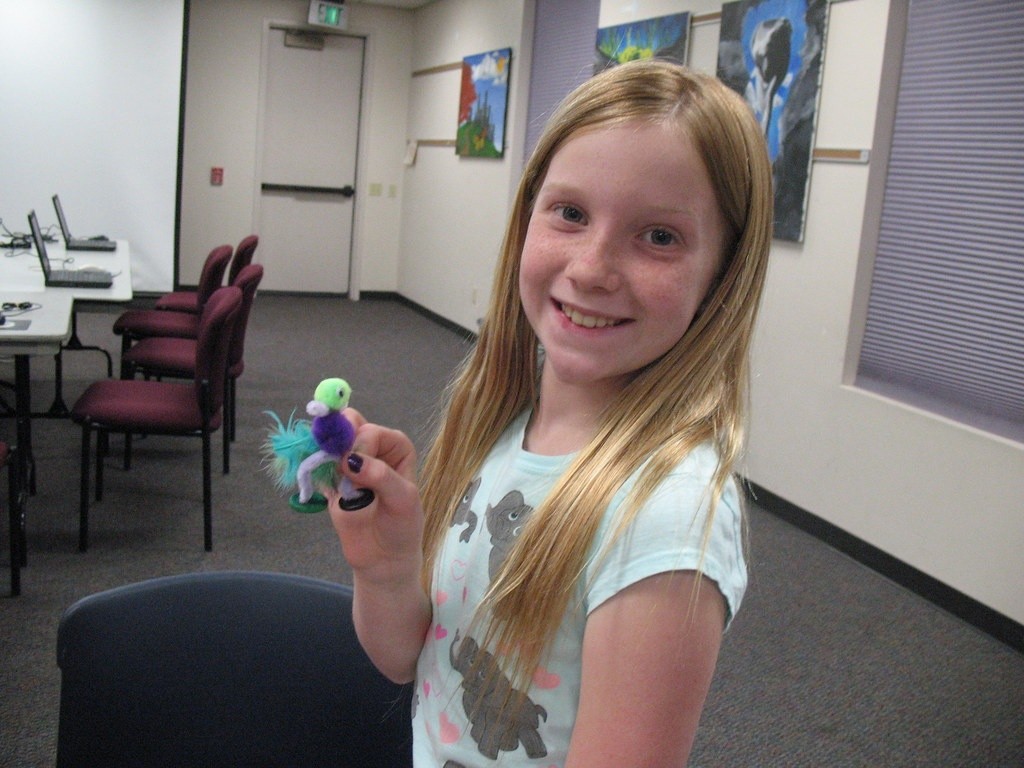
left=0, top=232, right=134, bottom=588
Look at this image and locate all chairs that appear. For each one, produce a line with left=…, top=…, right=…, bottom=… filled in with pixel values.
left=71, top=288, right=242, bottom=553
left=155, top=234, right=258, bottom=312
left=114, top=244, right=233, bottom=382
left=121, top=264, right=264, bottom=470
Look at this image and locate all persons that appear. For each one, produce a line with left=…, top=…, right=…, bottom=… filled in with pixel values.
left=325, top=59, right=774, bottom=768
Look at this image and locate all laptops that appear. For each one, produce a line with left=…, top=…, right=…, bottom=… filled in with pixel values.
left=53, top=194, right=117, bottom=251
left=28, top=209, right=113, bottom=289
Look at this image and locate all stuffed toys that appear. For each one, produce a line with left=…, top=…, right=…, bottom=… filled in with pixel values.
left=273, top=378, right=374, bottom=514
